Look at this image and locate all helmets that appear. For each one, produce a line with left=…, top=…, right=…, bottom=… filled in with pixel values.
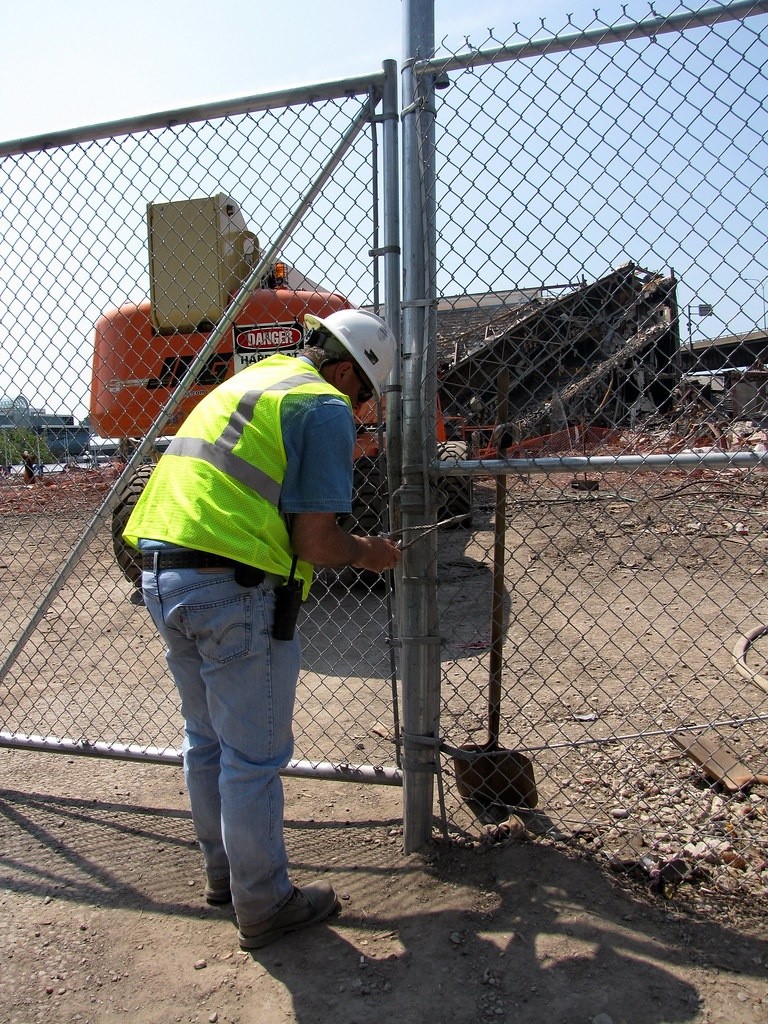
left=303, top=308, right=398, bottom=402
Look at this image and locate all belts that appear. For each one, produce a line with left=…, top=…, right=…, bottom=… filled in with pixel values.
left=140, top=547, right=239, bottom=570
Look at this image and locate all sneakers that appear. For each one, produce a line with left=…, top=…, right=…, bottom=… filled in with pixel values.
left=237, top=879, right=338, bottom=948
left=204, top=878, right=232, bottom=901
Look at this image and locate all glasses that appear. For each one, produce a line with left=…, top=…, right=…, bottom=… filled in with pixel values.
left=319, top=356, right=374, bottom=404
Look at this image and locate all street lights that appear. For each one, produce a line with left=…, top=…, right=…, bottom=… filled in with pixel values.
left=744, top=278, right=766, bottom=329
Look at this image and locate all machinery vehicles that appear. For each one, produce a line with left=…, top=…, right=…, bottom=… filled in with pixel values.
left=91, top=194, right=473, bottom=589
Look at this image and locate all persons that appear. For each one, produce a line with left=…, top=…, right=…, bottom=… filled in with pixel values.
left=137, top=308, right=403, bottom=950
